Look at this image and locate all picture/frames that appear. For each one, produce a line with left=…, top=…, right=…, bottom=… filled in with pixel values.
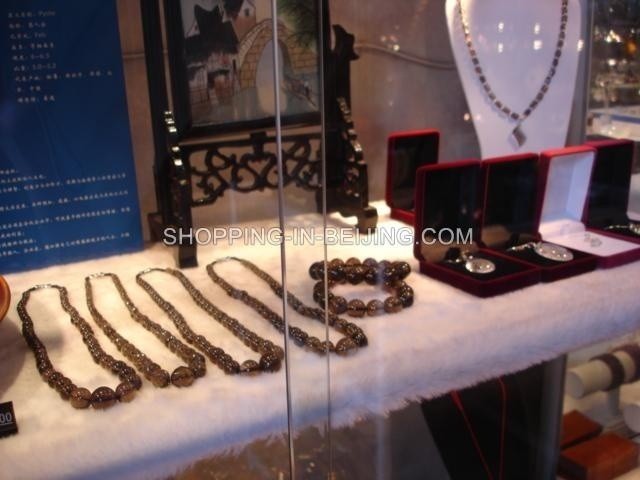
left=141, top=1, right=334, bottom=141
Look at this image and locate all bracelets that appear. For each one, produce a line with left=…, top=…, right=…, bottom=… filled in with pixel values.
left=310, top=276, right=414, bottom=319
left=307, top=257, right=414, bottom=282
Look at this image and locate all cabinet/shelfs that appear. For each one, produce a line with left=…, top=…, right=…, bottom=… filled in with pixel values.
left=1, top=198, right=640, bottom=480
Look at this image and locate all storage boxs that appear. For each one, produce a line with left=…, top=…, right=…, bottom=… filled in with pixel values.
left=384, top=131, right=640, bottom=298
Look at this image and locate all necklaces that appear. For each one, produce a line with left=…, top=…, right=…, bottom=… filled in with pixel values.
left=505, top=237, right=575, bottom=264
left=134, top=266, right=284, bottom=377
left=14, top=280, right=145, bottom=413
left=83, top=270, right=208, bottom=389
left=605, top=218, right=640, bottom=239
left=452, top=0, right=571, bottom=147
left=444, top=243, right=497, bottom=277
left=567, top=231, right=603, bottom=248
left=206, top=255, right=371, bottom=358
